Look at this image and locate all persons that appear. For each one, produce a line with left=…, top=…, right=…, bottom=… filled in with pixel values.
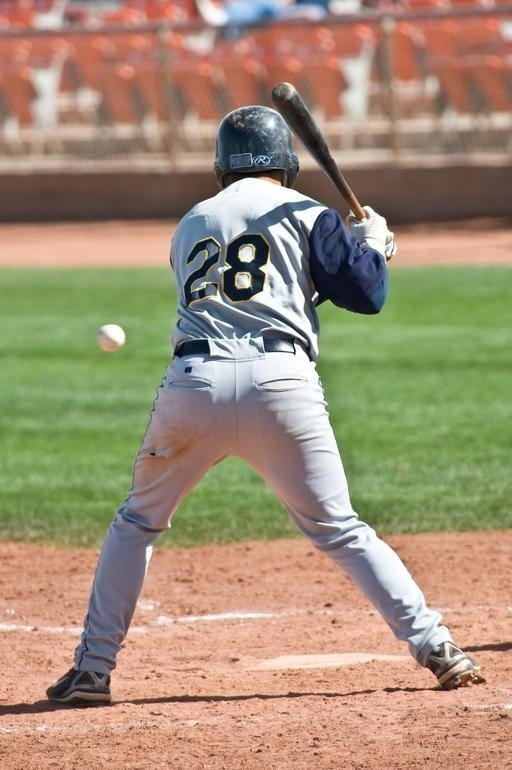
left=44, top=103, right=487, bottom=703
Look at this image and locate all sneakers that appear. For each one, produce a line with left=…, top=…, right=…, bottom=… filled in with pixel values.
left=46, top=667, right=112, bottom=704
left=427, top=640, right=482, bottom=689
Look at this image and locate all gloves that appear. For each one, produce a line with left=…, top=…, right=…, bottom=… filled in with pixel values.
left=347, top=205, right=398, bottom=265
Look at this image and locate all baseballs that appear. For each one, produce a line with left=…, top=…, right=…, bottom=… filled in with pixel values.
left=97, top=324, right=126, bottom=353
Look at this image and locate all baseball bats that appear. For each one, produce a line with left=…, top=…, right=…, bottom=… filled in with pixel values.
left=272, top=82, right=393, bottom=261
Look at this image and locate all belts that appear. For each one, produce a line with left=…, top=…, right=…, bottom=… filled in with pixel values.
left=177, top=339, right=294, bottom=355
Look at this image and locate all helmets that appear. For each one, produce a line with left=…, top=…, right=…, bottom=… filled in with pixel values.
left=213, top=106, right=300, bottom=189
